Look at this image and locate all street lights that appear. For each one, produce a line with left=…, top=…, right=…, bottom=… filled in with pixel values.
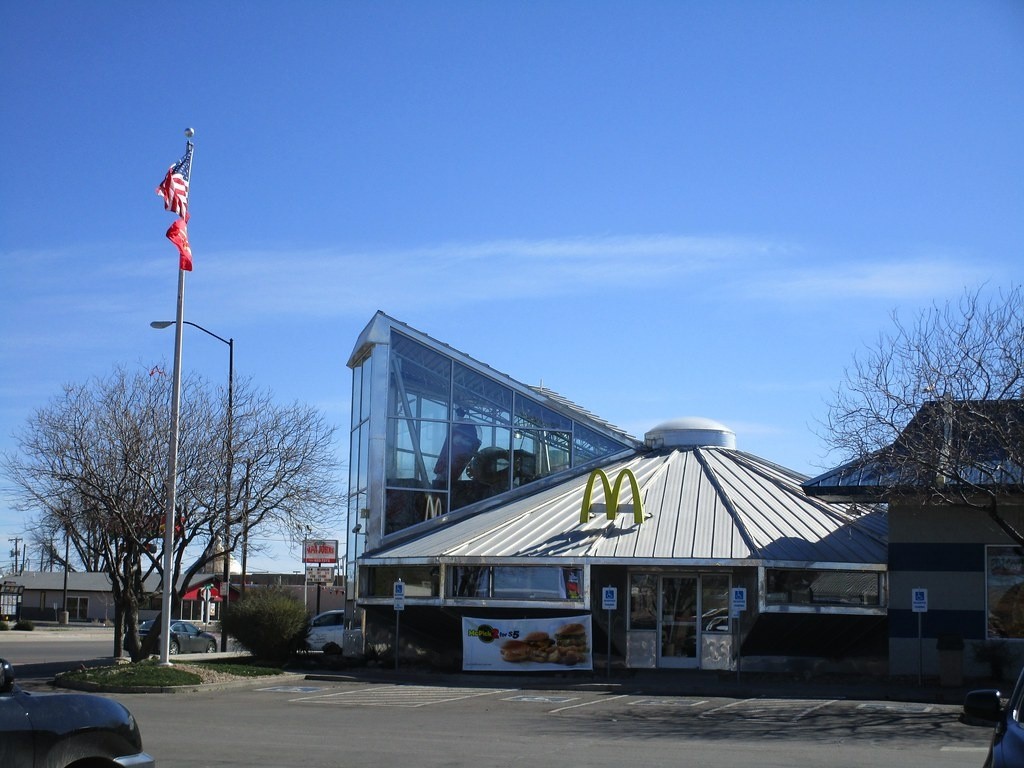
left=297, top=523, right=313, bottom=611
left=149, top=320, right=235, bottom=653
left=59, top=498, right=73, bottom=624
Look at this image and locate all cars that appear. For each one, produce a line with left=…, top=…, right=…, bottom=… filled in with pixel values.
left=963, top=665, right=1024, bottom=768
left=301, top=609, right=362, bottom=658
left=0, top=658, right=156, bottom=768
left=122, top=619, right=218, bottom=659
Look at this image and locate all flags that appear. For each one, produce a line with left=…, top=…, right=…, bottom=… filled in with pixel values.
left=166, top=213, right=193, bottom=272
left=154, top=150, right=192, bottom=219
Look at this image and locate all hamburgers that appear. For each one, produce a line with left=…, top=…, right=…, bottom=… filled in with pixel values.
left=500, top=631, right=555, bottom=661
left=554, top=623, right=587, bottom=654
